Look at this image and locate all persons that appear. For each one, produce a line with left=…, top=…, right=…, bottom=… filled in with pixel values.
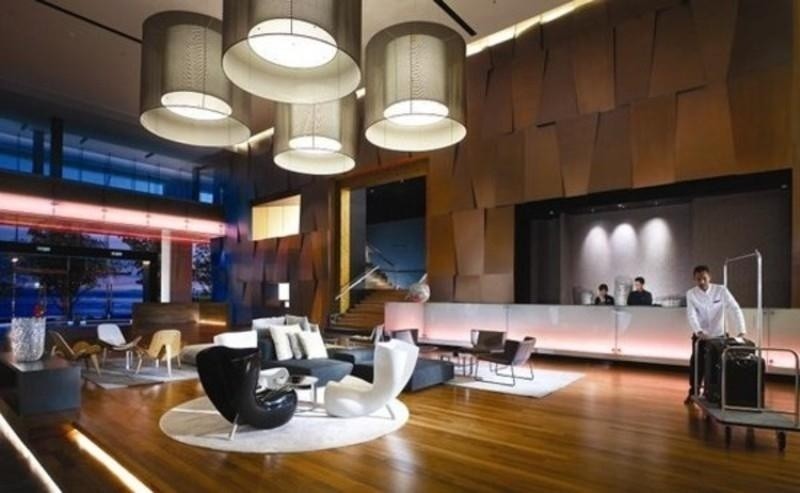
left=626, top=276, right=653, bottom=306
left=594, top=283, right=614, bottom=305
left=683, top=265, right=746, bottom=406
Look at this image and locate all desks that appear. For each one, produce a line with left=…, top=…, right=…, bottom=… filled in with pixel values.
left=272, top=374, right=319, bottom=411
left=447, top=354, right=475, bottom=379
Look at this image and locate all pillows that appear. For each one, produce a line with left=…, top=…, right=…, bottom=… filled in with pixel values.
left=297, top=332, right=328, bottom=360
left=286, top=331, right=304, bottom=360
left=286, top=315, right=309, bottom=331
left=269, top=323, right=302, bottom=361
left=309, top=323, right=320, bottom=332
left=251, top=317, right=285, bottom=329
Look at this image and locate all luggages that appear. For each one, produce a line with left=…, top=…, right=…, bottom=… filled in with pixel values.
left=704, top=336, right=765, bottom=412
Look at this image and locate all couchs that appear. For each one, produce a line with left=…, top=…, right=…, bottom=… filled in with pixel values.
left=327, top=346, right=454, bottom=392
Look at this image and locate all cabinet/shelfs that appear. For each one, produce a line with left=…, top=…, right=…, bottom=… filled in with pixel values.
left=0, top=351, right=82, bottom=417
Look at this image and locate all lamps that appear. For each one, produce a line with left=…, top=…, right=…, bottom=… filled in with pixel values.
left=363, top=21, right=469, bottom=153
left=139, top=10, right=251, bottom=148
left=220, top=16, right=362, bottom=104
left=273, top=91, right=361, bottom=176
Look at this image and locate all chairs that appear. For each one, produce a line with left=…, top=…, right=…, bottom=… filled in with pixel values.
left=259, top=338, right=353, bottom=386
left=477, top=336, right=535, bottom=385
left=195, top=345, right=296, bottom=440
left=49, top=330, right=101, bottom=375
left=453, top=329, right=507, bottom=371
left=97, top=323, right=142, bottom=370
left=324, top=338, right=420, bottom=419
left=132, top=329, right=183, bottom=376
left=392, top=328, right=441, bottom=357
left=212, top=330, right=290, bottom=387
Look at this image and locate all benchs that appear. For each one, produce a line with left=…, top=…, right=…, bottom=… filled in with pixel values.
left=132, top=299, right=232, bottom=343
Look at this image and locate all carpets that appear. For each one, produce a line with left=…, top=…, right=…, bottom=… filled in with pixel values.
left=427, top=355, right=583, bottom=400
left=158, top=396, right=409, bottom=454
left=81, top=357, right=200, bottom=390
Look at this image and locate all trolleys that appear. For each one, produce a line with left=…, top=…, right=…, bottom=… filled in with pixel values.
left=690, top=247, right=800, bottom=452
